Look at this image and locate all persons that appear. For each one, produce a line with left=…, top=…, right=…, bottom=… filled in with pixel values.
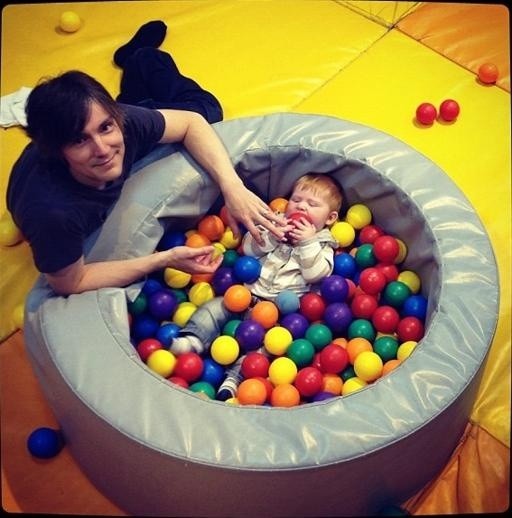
left=162, top=172, right=345, bottom=401
left=6, top=19, right=287, bottom=296
